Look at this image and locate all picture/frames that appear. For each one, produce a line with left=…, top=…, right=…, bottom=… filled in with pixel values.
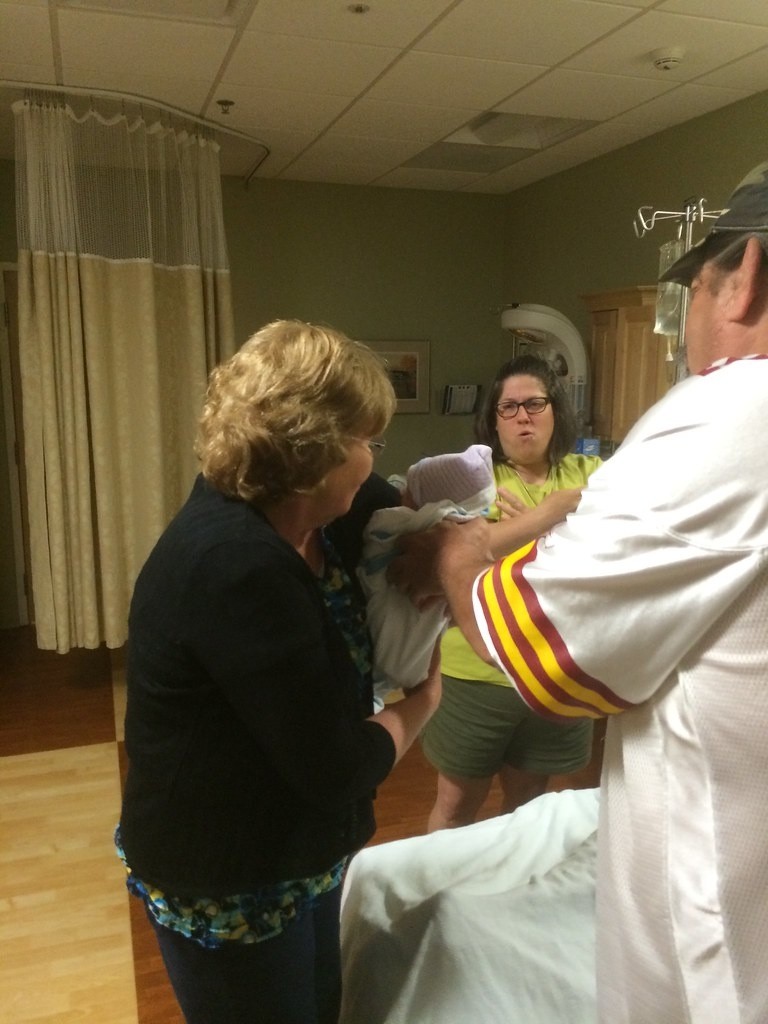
left=355, top=338, right=431, bottom=414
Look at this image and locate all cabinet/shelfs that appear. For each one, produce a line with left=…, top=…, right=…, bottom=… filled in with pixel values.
left=577, top=285, right=690, bottom=457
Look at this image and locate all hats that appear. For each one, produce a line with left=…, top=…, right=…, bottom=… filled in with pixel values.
left=657, top=160, right=768, bottom=289
left=407, top=444, right=495, bottom=508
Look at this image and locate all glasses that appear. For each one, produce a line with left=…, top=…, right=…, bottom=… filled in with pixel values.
left=344, top=436, right=386, bottom=457
left=494, top=397, right=549, bottom=418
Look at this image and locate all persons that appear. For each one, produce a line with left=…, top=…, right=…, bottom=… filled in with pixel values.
left=358, top=444, right=498, bottom=690
left=392, top=161, right=768, bottom=1024
left=113, top=317, right=442, bottom=1024
left=422, top=354, right=609, bottom=836
